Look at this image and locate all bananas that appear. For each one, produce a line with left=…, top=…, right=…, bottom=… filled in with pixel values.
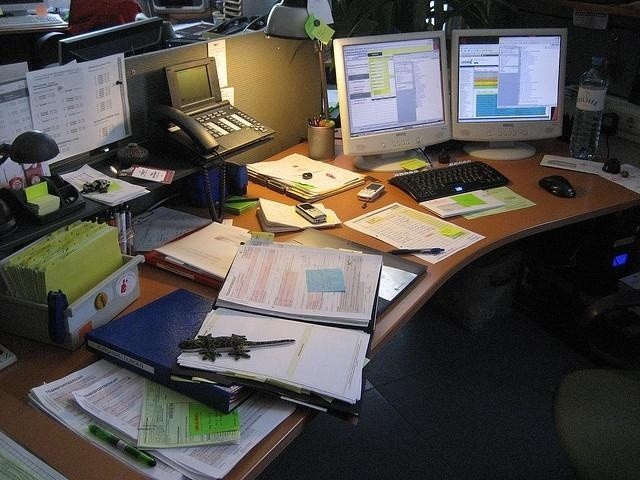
left=389, top=162, right=510, bottom=204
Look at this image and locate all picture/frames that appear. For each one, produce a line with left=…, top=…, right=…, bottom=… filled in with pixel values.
left=2, top=14, right=69, bottom=44
left=0, top=127, right=640, bottom=479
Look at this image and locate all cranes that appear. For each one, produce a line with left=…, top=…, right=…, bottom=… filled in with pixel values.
left=172, top=240, right=383, bottom=428
left=87, top=287, right=257, bottom=412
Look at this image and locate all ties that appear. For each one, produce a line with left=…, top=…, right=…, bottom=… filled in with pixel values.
left=58, top=17, right=166, bottom=68
left=334, top=30, right=452, bottom=173
left=452, top=26, right=569, bottom=160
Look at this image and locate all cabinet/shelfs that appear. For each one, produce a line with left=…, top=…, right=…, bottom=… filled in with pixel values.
left=568, top=55, right=609, bottom=160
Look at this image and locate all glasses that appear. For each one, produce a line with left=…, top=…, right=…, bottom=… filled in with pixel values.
left=0, top=131, right=61, bottom=167
left=264, top=0, right=331, bottom=121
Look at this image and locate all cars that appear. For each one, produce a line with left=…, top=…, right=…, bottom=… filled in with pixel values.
left=306, top=120, right=335, bottom=162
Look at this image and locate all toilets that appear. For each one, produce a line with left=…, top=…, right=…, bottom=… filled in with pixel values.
left=161, top=57, right=277, bottom=163
left=217, top=16, right=251, bottom=34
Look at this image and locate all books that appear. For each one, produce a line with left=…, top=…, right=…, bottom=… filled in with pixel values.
left=1, top=252, right=148, bottom=353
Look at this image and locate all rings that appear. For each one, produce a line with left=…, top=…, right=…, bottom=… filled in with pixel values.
left=308, top=115, right=322, bottom=127
left=91, top=424, right=156, bottom=467
left=387, top=248, right=446, bottom=254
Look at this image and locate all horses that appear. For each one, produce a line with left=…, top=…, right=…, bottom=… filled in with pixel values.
left=357, top=181, right=385, bottom=202
left=294, top=201, right=327, bottom=224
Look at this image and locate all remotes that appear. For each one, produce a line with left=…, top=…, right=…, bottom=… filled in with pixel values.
left=540, top=174, right=573, bottom=198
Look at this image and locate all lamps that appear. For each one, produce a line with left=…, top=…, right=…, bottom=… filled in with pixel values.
left=32, top=1, right=144, bottom=70
left=552, top=368, right=640, bottom=480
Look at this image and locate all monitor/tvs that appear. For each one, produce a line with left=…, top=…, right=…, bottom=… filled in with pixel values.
left=418, top=188, right=507, bottom=220
left=254, top=196, right=336, bottom=233
left=175, top=239, right=384, bottom=427
left=143, top=220, right=250, bottom=290
left=246, top=152, right=368, bottom=204
left=173, top=23, right=217, bottom=37
